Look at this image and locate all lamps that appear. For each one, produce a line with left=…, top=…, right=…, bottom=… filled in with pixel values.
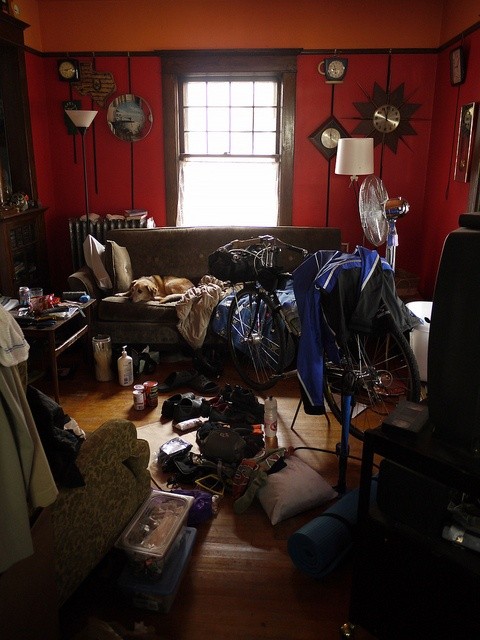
left=64, top=108, right=98, bottom=233
left=336, top=138, right=374, bottom=182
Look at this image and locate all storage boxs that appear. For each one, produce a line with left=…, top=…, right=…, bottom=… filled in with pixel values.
left=406, top=301, right=433, bottom=382
left=114, top=490, right=194, bottom=575
left=116, top=527, right=198, bottom=615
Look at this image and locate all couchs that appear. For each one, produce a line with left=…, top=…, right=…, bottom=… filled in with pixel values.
left=67, top=227, right=343, bottom=377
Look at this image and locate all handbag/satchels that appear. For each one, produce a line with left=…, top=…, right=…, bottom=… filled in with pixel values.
left=173, top=452, right=206, bottom=482
left=208, top=235, right=308, bottom=284
left=158, top=437, right=193, bottom=465
left=173, top=490, right=212, bottom=524
left=197, top=421, right=246, bottom=461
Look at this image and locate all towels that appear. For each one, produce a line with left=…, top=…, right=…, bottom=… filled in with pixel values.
left=83, top=234, right=112, bottom=290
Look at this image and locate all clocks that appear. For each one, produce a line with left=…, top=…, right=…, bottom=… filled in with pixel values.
left=352, top=83, right=421, bottom=154
left=57, top=57, right=80, bottom=82
left=322, top=56, right=347, bottom=81
left=448, top=46, right=463, bottom=86
left=309, top=115, right=351, bottom=160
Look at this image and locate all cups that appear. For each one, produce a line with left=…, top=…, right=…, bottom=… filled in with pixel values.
left=28, top=287, right=42, bottom=299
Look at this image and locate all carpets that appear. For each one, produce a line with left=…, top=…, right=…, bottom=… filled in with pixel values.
left=136, top=420, right=205, bottom=497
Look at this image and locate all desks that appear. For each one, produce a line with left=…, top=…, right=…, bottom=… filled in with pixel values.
left=22, top=298, right=97, bottom=406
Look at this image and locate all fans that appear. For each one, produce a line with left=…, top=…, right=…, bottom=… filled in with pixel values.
left=358, top=174, right=412, bottom=396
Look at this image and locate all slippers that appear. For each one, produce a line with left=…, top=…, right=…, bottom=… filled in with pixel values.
left=195, top=375, right=219, bottom=393
left=158, top=370, right=198, bottom=392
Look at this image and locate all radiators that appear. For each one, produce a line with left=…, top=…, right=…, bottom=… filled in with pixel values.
left=68, top=217, right=156, bottom=273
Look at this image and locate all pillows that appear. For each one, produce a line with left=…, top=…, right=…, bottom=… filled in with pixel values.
left=104, top=240, right=133, bottom=292
left=257, top=455, right=338, bottom=525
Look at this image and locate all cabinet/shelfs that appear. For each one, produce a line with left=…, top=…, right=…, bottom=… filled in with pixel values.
left=348, top=423, right=480, bottom=640
left=0, top=206, right=50, bottom=297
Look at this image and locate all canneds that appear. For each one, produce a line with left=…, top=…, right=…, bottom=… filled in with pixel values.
left=143, top=381, right=158, bottom=407
left=133, top=384, right=145, bottom=390
left=133, top=390, right=146, bottom=411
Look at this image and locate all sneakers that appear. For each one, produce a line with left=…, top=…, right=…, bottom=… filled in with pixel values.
left=210, top=403, right=256, bottom=421
left=163, top=392, right=195, bottom=418
left=200, top=394, right=223, bottom=416
left=231, top=384, right=264, bottom=415
left=175, top=398, right=202, bottom=420
left=221, top=384, right=238, bottom=401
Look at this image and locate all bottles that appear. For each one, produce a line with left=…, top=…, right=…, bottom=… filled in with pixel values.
left=263, top=395, right=278, bottom=437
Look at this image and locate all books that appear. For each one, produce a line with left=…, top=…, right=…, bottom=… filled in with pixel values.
left=126, top=215, right=148, bottom=220
left=126, top=210, right=148, bottom=217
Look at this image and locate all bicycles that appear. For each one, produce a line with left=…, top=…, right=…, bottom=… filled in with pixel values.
left=208, top=234, right=420, bottom=443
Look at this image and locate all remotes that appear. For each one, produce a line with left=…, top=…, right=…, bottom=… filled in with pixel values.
left=44, top=304, right=71, bottom=313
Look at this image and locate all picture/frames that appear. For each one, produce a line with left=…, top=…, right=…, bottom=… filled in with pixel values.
left=452, top=102, right=476, bottom=183
